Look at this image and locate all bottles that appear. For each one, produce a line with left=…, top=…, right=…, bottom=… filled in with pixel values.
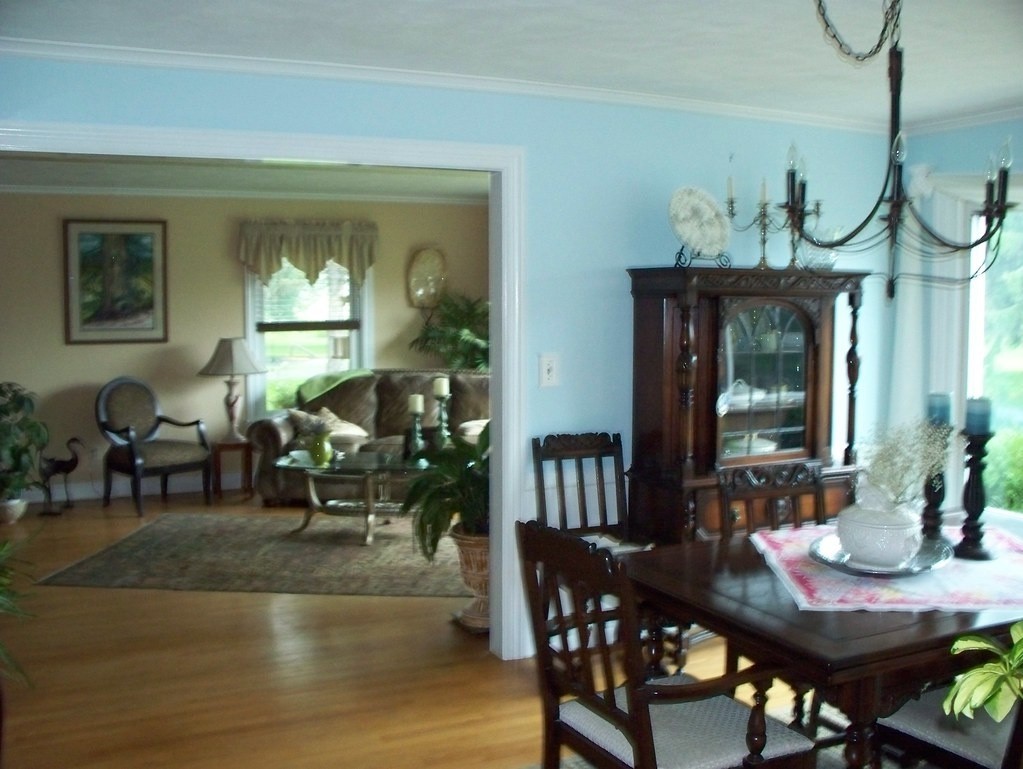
left=794, top=366, right=801, bottom=391
left=725, top=329, right=758, bottom=352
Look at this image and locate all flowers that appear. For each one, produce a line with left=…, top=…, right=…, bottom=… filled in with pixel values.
left=849, top=412, right=969, bottom=511
left=296, top=416, right=329, bottom=430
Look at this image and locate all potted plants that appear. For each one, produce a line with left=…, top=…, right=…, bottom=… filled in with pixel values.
left=0, top=383, right=48, bottom=524
left=400, top=421, right=489, bottom=629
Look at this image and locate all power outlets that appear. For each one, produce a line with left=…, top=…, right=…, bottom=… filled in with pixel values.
left=539, top=354, right=559, bottom=387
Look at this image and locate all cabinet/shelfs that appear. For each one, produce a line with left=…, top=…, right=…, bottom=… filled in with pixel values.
left=624, top=267, right=871, bottom=649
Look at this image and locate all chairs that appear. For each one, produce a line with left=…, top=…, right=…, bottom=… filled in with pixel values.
left=513, top=432, right=1023, bottom=769
left=95, top=377, right=213, bottom=516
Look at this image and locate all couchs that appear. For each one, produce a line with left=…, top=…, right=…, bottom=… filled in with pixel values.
left=246, top=369, right=490, bottom=510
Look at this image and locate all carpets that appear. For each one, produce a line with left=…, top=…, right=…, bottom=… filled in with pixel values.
left=34, top=512, right=477, bottom=597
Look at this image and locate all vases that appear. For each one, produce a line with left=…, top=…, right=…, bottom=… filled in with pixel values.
left=837, top=506, right=923, bottom=571
left=296, top=430, right=334, bottom=469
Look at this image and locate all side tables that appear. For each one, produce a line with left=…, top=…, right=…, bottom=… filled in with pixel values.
left=210, top=442, right=253, bottom=507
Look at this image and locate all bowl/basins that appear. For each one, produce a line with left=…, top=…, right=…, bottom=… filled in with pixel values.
left=760, top=332, right=801, bottom=352
left=796, top=227, right=844, bottom=273
left=733, top=387, right=764, bottom=406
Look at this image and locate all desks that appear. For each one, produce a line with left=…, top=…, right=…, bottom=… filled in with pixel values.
left=621, top=506, right=1023, bottom=769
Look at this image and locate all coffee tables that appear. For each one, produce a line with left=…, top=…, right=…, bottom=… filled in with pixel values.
left=272, top=453, right=435, bottom=546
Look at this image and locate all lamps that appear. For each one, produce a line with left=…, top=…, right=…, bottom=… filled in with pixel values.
left=198, top=337, right=269, bottom=443
left=776, top=0, right=1019, bottom=300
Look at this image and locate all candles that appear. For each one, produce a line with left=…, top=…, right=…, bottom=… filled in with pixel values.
left=727, top=175, right=767, bottom=200
left=928, top=392, right=992, bottom=433
left=408, top=378, right=449, bottom=412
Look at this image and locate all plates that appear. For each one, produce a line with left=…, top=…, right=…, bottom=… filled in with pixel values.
left=669, top=185, right=730, bottom=257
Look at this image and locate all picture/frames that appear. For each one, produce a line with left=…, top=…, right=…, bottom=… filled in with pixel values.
left=62, top=219, right=168, bottom=344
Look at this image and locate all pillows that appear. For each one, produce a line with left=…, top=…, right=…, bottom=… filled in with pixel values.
left=287, top=406, right=370, bottom=436
